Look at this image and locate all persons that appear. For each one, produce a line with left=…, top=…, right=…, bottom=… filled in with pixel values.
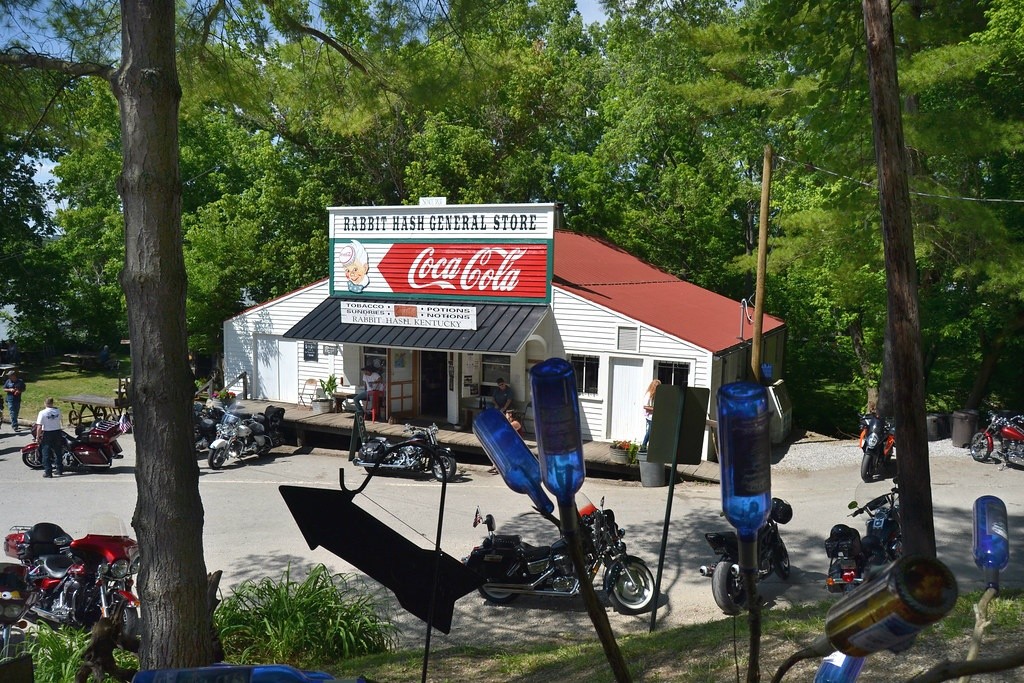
left=491, top=378, right=523, bottom=471
left=36, top=397, right=62, bottom=477
left=640, top=379, right=662, bottom=445
left=6, top=339, right=17, bottom=359
left=100, top=345, right=114, bottom=371
left=353, top=366, right=382, bottom=420
left=3, top=370, right=26, bottom=432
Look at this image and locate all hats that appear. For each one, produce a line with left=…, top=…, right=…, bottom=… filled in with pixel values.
left=5, top=370, right=15, bottom=375
left=360, top=366, right=372, bottom=372
left=496, top=378, right=505, bottom=385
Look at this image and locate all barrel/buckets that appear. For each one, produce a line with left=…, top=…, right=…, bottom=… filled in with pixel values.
left=926, top=413, right=946, bottom=440
left=952, top=409, right=978, bottom=448
left=638, top=449, right=666, bottom=487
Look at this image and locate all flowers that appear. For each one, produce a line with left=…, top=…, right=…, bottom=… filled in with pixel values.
left=210, top=388, right=236, bottom=399
left=613, top=440, right=631, bottom=451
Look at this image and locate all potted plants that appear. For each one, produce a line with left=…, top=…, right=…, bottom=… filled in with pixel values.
left=311, top=375, right=339, bottom=412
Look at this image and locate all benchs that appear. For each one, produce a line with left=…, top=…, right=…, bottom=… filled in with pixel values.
left=332, top=392, right=385, bottom=418
left=462, top=401, right=532, bottom=435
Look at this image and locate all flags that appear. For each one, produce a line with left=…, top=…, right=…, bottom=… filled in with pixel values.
left=118, top=415, right=132, bottom=433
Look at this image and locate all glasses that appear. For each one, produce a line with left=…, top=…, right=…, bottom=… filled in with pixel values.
left=499, top=383, right=503, bottom=386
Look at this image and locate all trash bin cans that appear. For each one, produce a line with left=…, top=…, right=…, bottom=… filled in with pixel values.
left=638, top=450, right=665, bottom=487
left=950, top=408, right=979, bottom=448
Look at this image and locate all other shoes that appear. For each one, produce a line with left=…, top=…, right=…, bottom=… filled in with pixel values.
left=43, top=474, right=52, bottom=478
left=488, top=468, right=498, bottom=474
left=54, top=472, right=62, bottom=475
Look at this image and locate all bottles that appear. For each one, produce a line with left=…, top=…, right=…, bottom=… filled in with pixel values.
left=717, top=382, right=773, bottom=578
left=972, top=495, right=1011, bottom=591
left=471, top=409, right=556, bottom=514
left=131, top=663, right=368, bottom=683
left=529, top=358, right=586, bottom=535
left=810, top=553, right=959, bottom=683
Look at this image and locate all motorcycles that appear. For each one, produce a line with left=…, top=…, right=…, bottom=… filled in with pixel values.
left=191, top=402, right=226, bottom=456
left=969, top=402, right=1024, bottom=471
left=201, top=399, right=276, bottom=471
left=352, top=422, right=457, bottom=482
left=20, top=415, right=125, bottom=475
left=0, top=558, right=46, bottom=653
left=4, top=523, right=141, bottom=640
left=824, top=476, right=904, bottom=597
left=855, top=410, right=897, bottom=483
left=699, top=497, right=794, bottom=616
left=461, top=489, right=655, bottom=615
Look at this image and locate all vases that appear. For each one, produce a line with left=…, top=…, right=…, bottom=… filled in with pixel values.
left=609, top=446, right=628, bottom=464
left=213, top=399, right=236, bottom=409
left=637, top=450, right=666, bottom=487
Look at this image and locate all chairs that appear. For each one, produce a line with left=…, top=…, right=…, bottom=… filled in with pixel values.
left=296, top=379, right=318, bottom=412
